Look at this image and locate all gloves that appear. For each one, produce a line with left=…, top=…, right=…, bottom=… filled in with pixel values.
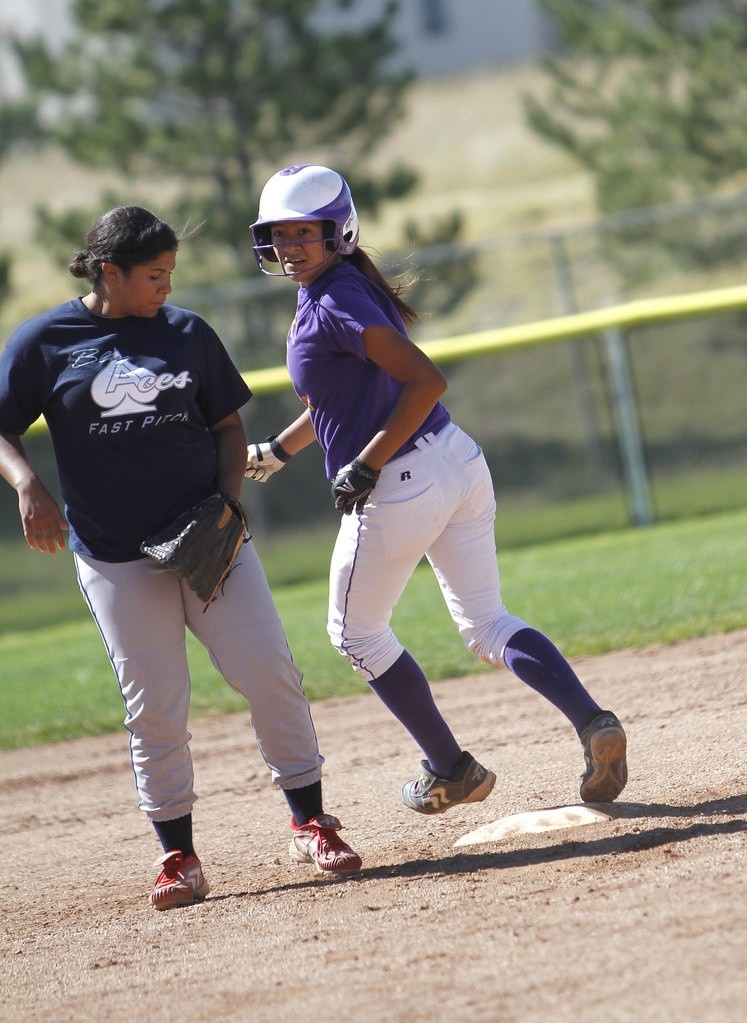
left=332, top=456, right=381, bottom=516
left=244, top=435, right=293, bottom=483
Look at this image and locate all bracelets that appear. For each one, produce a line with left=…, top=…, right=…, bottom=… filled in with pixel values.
left=266, top=434, right=295, bottom=463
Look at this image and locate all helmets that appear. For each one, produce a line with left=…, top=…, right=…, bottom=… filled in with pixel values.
left=249, top=164, right=360, bottom=255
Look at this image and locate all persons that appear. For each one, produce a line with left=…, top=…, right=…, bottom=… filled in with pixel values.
left=245, top=164, right=629, bottom=814
left=0, top=207, right=362, bottom=906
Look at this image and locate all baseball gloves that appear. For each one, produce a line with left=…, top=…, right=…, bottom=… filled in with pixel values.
left=140, top=491, right=246, bottom=605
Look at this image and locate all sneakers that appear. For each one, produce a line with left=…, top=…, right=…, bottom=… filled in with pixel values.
left=289, top=812, right=362, bottom=875
left=401, top=751, right=496, bottom=814
left=151, top=850, right=210, bottom=910
left=578, top=710, right=627, bottom=802
